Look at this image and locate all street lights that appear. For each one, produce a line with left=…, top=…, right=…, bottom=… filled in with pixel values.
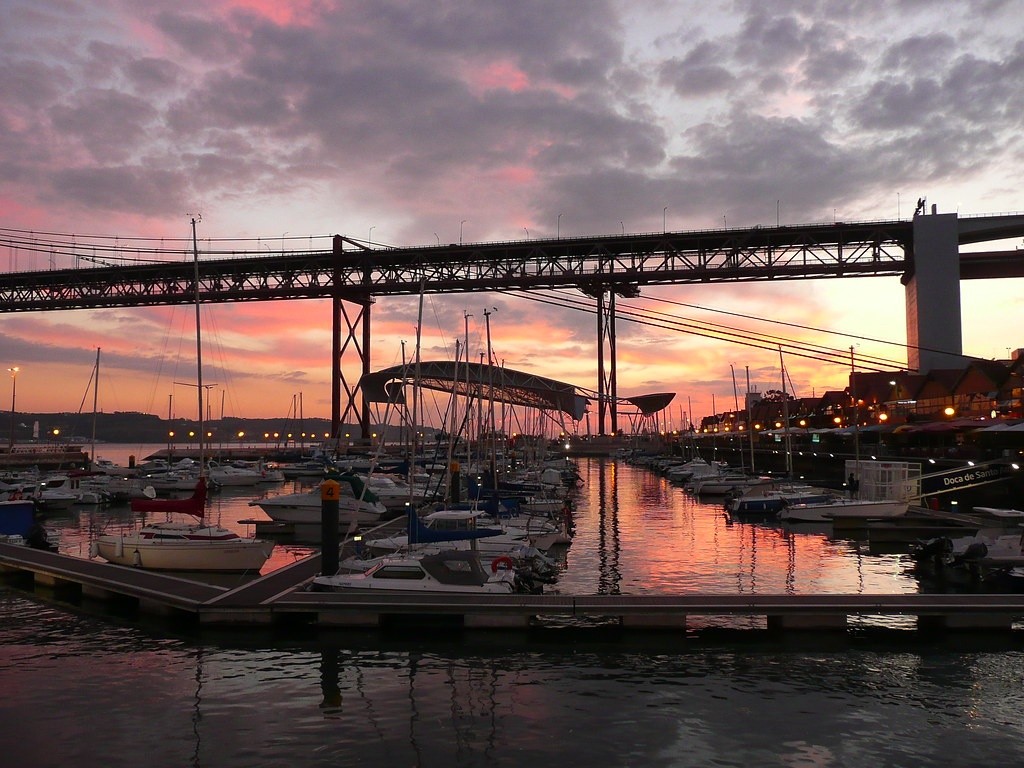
left=460, top=220, right=466, bottom=246
left=777, top=200, right=779, bottom=228
left=558, top=213, right=562, bottom=241
left=434, top=233, right=439, bottom=247
left=369, top=226, right=375, bottom=249
left=664, top=207, right=667, bottom=234
left=621, top=222, right=624, bottom=235
left=282, top=231, right=289, bottom=256
left=898, top=192, right=900, bottom=221
left=723, top=216, right=726, bottom=231
left=834, top=208, right=836, bottom=224
left=524, top=227, right=528, bottom=242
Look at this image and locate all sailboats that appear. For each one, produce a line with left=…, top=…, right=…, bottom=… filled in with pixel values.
left=0, top=306, right=1024, bottom=598
left=90, top=208, right=279, bottom=575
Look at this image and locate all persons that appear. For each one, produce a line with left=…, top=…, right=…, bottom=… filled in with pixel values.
left=848, top=473, right=856, bottom=498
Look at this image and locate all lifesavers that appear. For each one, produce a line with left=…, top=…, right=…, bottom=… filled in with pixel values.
left=492, top=557, right=512, bottom=572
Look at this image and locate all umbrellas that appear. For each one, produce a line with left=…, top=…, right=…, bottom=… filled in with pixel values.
left=760, top=422, right=1024, bottom=457
